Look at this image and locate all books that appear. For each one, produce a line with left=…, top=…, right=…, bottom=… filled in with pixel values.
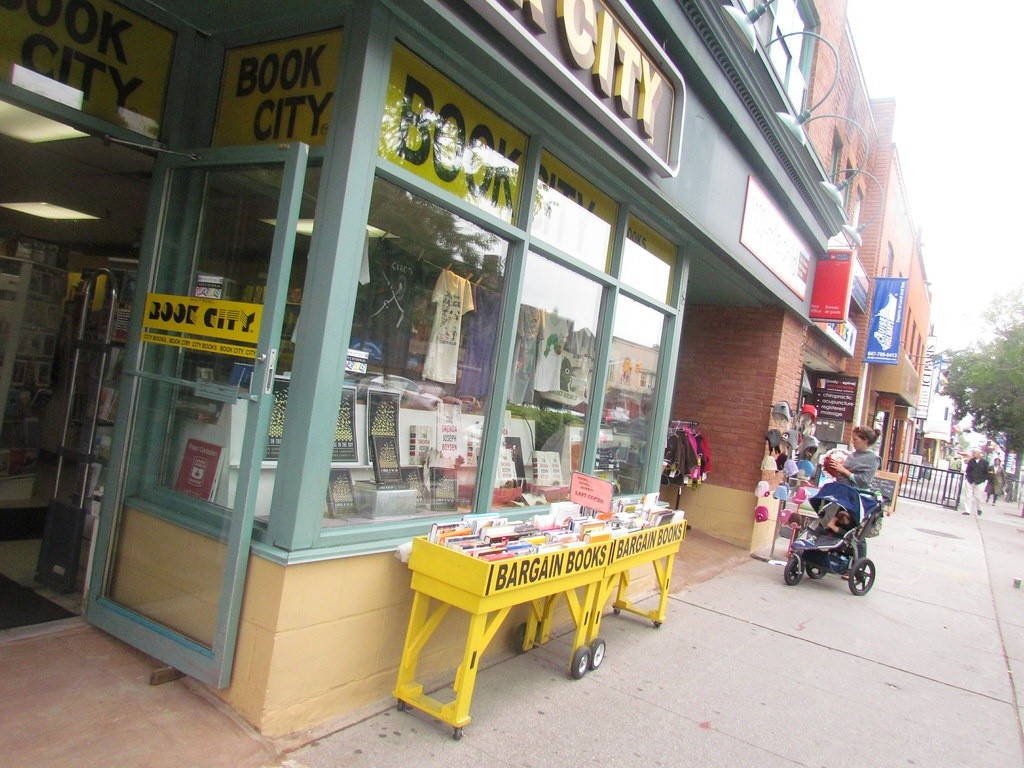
left=427, top=492, right=685, bottom=563
left=71, top=346, right=122, bottom=506
left=176, top=438, right=225, bottom=502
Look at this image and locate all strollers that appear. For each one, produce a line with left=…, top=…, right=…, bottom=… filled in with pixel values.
left=783, top=480, right=892, bottom=596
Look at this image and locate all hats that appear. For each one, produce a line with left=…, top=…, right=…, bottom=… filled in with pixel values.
left=798, top=460, right=815, bottom=478
left=773, top=486, right=788, bottom=500
left=824, top=456, right=836, bottom=477
left=799, top=404, right=817, bottom=424
left=779, top=509, right=793, bottom=526
left=754, top=506, right=769, bottom=522
left=792, top=488, right=807, bottom=504
left=776, top=453, right=787, bottom=472
left=783, top=460, right=797, bottom=477
left=760, top=455, right=779, bottom=472
left=754, top=481, right=770, bottom=498
left=763, top=428, right=818, bottom=460
left=772, top=401, right=789, bottom=422
left=788, top=512, right=801, bottom=528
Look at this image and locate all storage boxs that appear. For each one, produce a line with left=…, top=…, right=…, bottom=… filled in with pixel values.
left=195, top=274, right=237, bottom=300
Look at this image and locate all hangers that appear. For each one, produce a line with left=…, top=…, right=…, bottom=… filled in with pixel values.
left=667, top=420, right=700, bottom=439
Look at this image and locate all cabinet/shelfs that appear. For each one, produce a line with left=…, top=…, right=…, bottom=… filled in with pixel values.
left=79, top=257, right=138, bottom=571
left=0, top=256, right=71, bottom=500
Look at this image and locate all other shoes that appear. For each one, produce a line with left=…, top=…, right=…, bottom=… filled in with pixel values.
left=992, top=502, right=996, bottom=506
left=978, top=510, right=982, bottom=515
left=986, top=498, right=989, bottom=502
left=963, top=512, right=969, bottom=515
left=841, top=573, right=856, bottom=580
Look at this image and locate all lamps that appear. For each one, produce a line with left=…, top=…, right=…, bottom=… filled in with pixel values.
left=803, top=115, right=869, bottom=208
left=721, top=0, right=775, bottom=55
left=830, top=169, right=884, bottom=247
left=765, top=31, right=840, bottom=146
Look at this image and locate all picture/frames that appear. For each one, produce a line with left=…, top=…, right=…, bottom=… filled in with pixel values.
left=329, top=385, right=361, bottom=466
left=430, top=467, right=458, bottom=511
left=326, top=467, right=359, bottom=518
left=367, top=388, right=401, bottom=466
left=249, top=372, right=290, bottom=466
left=371, top=434, right=402, bottom=484
left=401, top=466, right=426, bottom=507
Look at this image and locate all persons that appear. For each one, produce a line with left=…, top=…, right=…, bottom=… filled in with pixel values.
left=829, top=426, right=882, bottom=580
left=794, top=510, right=854, bottom=547
left=949, top=449, right=1007, bottom=515
